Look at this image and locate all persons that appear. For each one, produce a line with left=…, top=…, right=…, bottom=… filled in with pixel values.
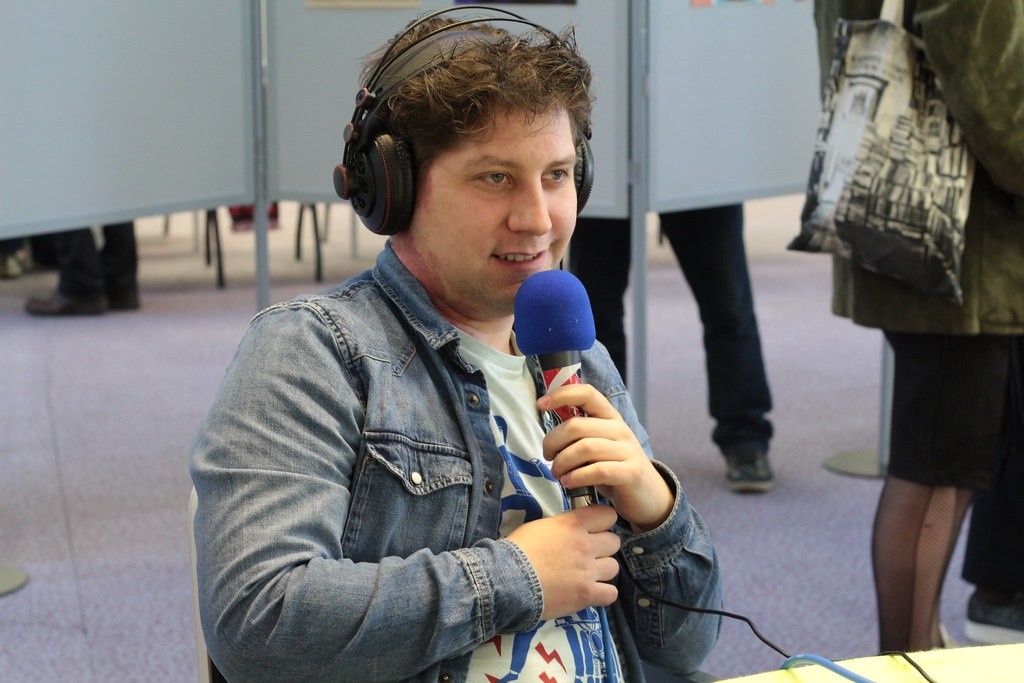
left=190, top=18, right=722, bottom=683
left=564, top=202, right=775, bottom=493
left=786, top=0, right=1024, bottom=655
left=25, top=220, right=141, bottom=319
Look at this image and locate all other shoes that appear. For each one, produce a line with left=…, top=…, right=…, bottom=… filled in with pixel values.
left=724, top=448, right=775, bottom=492
left=26, top=291, right=109, bottom=316
left=963, top=588, right=1024, bottom=645
left=110, top=292, right=139, bottom=309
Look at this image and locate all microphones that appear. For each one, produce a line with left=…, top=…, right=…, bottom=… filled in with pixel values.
left=512, top=268, right=595, bottom=513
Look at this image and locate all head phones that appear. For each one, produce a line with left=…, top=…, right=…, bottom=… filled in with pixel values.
left=331, top=6, right=596, bottom=236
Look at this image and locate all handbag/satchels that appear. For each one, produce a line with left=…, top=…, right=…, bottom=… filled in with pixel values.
left=786, top=0, right=979, bottom=307
